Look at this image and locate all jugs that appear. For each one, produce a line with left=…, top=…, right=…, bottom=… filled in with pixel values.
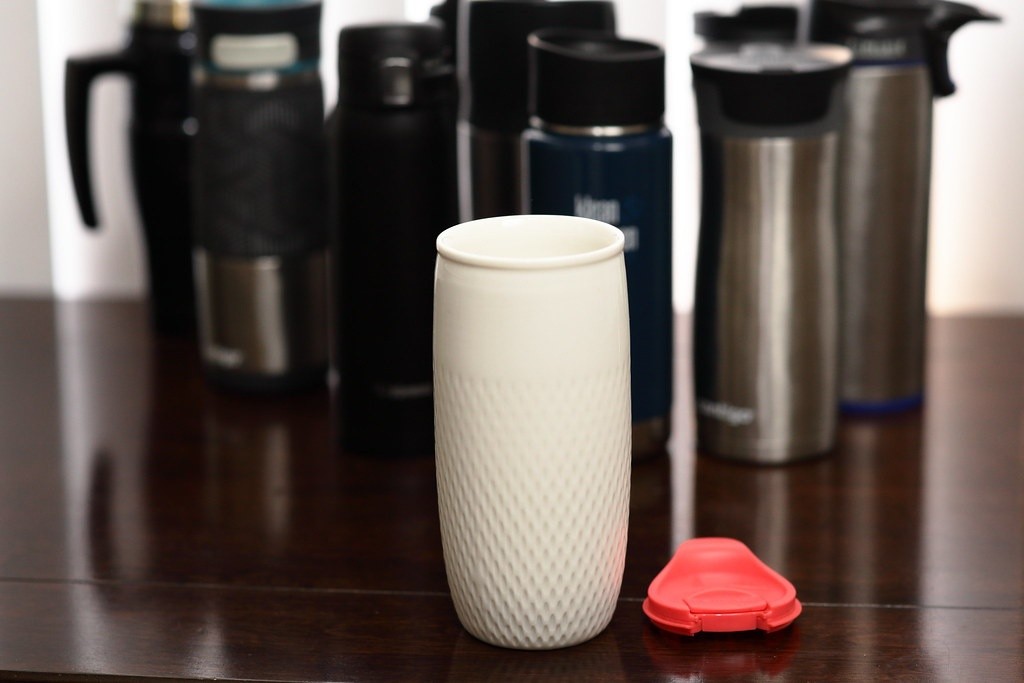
left=61, top=1, right=194, bottom=323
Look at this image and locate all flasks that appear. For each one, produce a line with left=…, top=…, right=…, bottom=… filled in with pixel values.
left=322, top=21, right=457, bottom=399
left=691, top=3, right=802, bottom=56
left=457, top=0, right=617, bottom=224
left=182, top=0, right=329, bottom=391
left=807, top=0, right=988, bottom=419
left=518, top=26, right=672, bottom=452
left=688, top=35, right=831, bottom=466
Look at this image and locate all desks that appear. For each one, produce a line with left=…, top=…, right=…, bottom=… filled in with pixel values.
left=0, top=294, right=1022, bottom=683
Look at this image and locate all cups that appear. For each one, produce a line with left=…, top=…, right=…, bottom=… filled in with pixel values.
left=433, top=212, right=633, bottom=648
